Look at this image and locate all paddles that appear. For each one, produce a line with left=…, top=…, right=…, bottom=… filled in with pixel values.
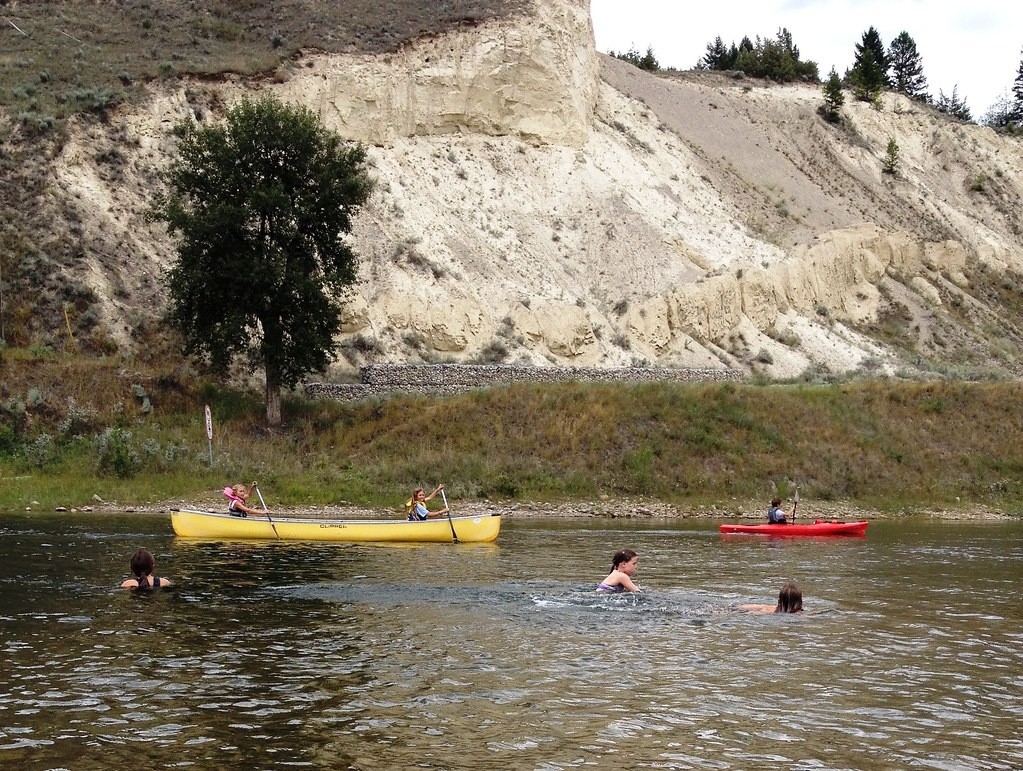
left=440, top=486, right=459, bottom=542
left=254, top=483, right=282, bottom=540
left=792, top=489, right=799, bottom=522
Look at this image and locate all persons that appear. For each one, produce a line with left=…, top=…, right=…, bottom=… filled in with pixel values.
left=122, top=549, right=170, bottom=587
left=769, top=584, right=804, bottom=613
left=409, top=484, right=450, bottom=521
left=768, top=499, right=797, bottom=523
left=229, top=480, right=270, bottom=517
left=596, top=549, right=640, bottom=593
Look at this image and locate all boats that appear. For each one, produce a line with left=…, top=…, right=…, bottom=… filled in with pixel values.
left=718, top=521, right=869, bottom=532
left=170, top=509, right=500, bottom=546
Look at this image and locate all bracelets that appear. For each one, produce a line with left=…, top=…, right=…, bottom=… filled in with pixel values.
left=438, top=511, right=442, bottom=515
